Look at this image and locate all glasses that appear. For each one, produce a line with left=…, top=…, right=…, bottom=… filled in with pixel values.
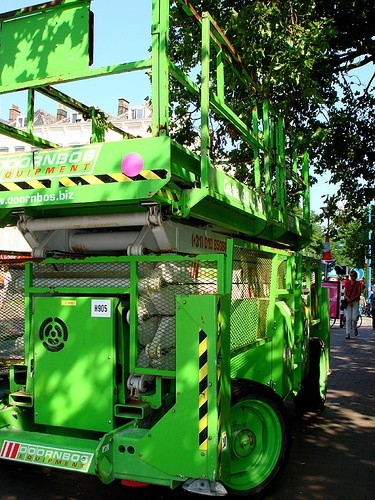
left=350, top=275, right=355, bottom=277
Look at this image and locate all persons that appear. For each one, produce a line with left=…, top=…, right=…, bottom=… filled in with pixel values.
left=371, top=293, right=375, bottom=327
left=344, top=271, right=361, bottom=339
left=359, top=293, right=365, bottom=316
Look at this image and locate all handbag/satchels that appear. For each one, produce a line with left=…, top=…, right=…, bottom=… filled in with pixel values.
left=340, top=298, right=349, bottom=311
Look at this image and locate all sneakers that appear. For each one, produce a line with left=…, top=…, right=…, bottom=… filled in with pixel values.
left=345, top=330, right=358, bottom=340
